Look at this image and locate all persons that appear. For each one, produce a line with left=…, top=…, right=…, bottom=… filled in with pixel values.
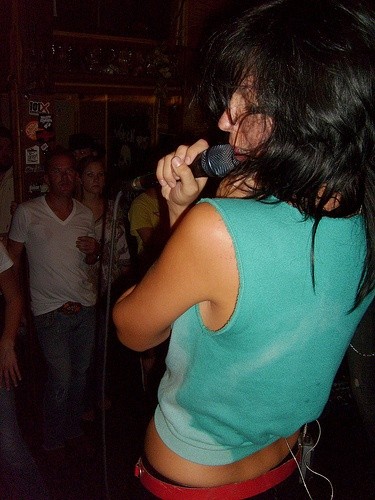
left=128, top=151, right=170, bottom=404
left=76, top=155, right=134, bottom=435
left=5, top=146, right=101, bottom=477
left=0, top=125, right=17, bottom=239
left=113, top=1, right=375, bottom=500
left=1, top=240, right=50, bottom=500
left=68, top=133, right=96, bottom=165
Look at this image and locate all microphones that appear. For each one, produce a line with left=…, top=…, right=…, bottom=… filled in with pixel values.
left=130, top=144, right=242, bottom=189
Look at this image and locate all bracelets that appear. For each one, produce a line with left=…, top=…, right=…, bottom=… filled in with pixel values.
left=95, top=253, right=100, bottom=260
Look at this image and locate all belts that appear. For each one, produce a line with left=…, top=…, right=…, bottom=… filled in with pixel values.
left=135, top=447, right=302, bottom=500
left=57, top=302, right=96, bottom=315
